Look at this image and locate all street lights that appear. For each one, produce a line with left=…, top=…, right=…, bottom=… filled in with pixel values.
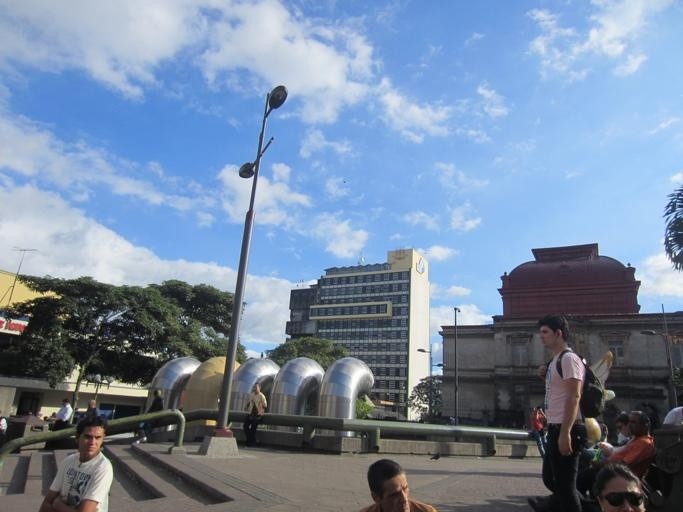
left=84, top=375, right=113, bottom=401
left=212, top=83, right=289, bottom=435
left=453, top=306, right=462, bottom=425
left=384, top=386, right=405, bottom=420
left=639, top=302, right=683, bottom=409
left=417, top=346, right=446, bottom=420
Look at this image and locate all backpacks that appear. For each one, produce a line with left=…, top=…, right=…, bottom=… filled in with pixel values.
left=556, top=348, right=605, bottom=420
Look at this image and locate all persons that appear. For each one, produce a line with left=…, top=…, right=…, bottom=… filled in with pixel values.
left=36, top=414, right=113, bottom=512
left=356, top=457, right=436, bottom=512
left=525, top=402, right=683, bottom=512
left=0, top=395, right=100, bottom=444
left=241, top=381, right=268, bottom=447
left=130, top=388, right=165, bottom=446
left=535, top=310, right=587, bottom=511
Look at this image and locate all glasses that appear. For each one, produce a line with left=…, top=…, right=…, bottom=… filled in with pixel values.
left=598, top=491, right=647, bottom=507
